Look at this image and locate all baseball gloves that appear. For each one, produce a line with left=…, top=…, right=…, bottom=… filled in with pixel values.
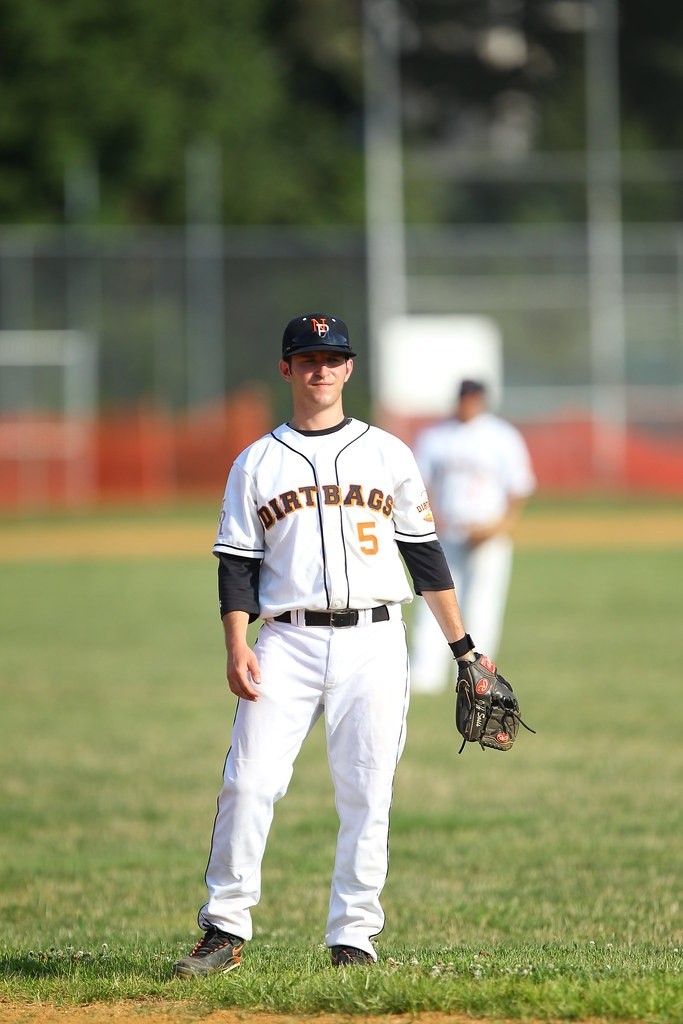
left=454, top=651, right=537, bottom=754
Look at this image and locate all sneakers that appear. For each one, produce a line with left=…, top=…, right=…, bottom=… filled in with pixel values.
left=329, top=942, right=380, bottom=970
left=173, top=916, right=244, bottom=981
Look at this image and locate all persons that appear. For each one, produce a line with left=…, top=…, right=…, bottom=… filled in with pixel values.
left=172, top=315, right=520, bottom=979
left=404, top=379, right=537, bottom=695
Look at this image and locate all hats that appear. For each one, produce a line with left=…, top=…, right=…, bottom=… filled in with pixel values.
left=461, top=380, right=489, bottom=397
left=279, top=311, right=358, bottom=364
